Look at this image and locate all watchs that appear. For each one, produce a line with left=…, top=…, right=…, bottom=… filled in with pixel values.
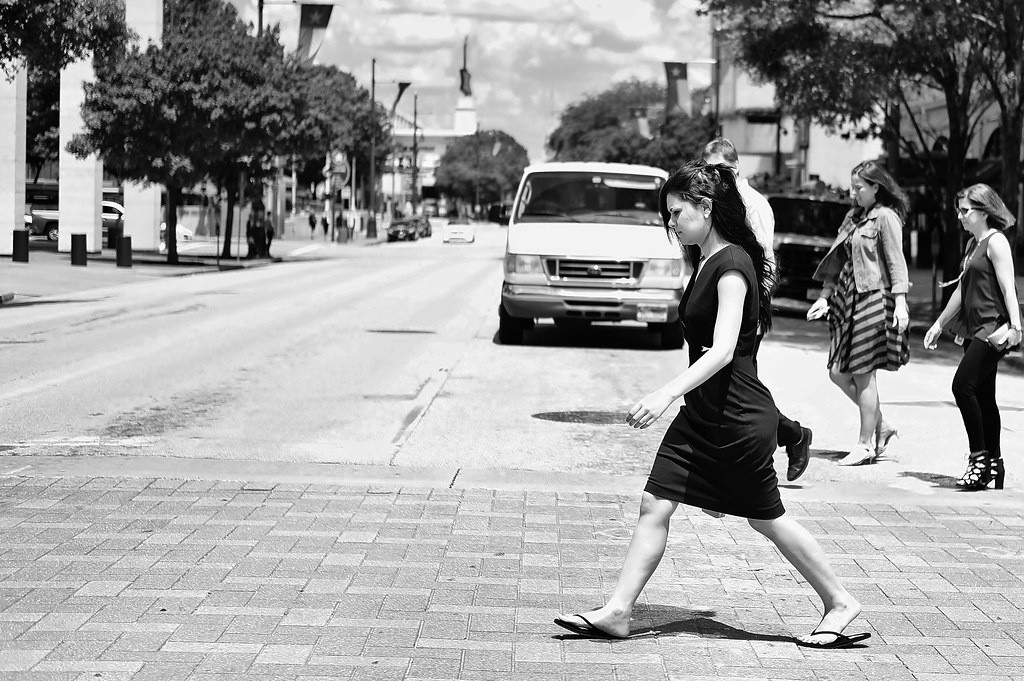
left=1011, top=322, right=1021, bottom=331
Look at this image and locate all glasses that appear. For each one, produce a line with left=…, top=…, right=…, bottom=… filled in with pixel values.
left=956, top=207, right=987, bottom=215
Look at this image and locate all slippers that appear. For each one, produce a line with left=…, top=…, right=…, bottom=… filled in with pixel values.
left=553, top=613, right=627, bottom=639
left=797, top=629, right=871, bottom=648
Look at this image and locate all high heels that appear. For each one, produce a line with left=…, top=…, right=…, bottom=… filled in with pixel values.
left=837, top=445, right=876, bottom=466
left=955, top=451, right=1005, bottom=491
left=874, top=426, right=900, bottom=458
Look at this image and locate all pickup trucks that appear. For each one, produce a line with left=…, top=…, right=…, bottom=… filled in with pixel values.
left=32, top=201, right=125, bottom=241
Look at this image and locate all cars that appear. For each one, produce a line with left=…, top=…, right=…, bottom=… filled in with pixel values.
left=386, top=215, right=432, bottom=242
left=25, top=215, right=33, bottom=236
left=443, top=218, right=475, bottom=244
left=488, top=203, right=515, bottom=225
left=175, top=224, right=194, bottom=242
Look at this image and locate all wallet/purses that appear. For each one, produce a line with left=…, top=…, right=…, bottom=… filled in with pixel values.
left=987, top=321, right=1011, bottom=352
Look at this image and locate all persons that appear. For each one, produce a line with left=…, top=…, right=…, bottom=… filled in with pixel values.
left=554, top=164, right=872, bottom=649
left=922, top=183, right=1023, bottom=491
left=703, top=136, right=813, bottom=481
left=309, top=211, right=316, bottom=240
left=336, top=213, right=343, bottom=242
left=806, top=161, right=909, bottom=466
left=247, top=212, right=274, bottom=259
left=320, top=212, right=330, bottom=241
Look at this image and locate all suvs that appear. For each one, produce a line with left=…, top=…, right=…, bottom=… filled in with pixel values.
left=764, top=192, right=854, bottom=302
left=487, top=160, right=692, bottom=351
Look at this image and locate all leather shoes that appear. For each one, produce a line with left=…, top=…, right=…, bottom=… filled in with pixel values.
left=786, top=427, right=812, bottom=481
